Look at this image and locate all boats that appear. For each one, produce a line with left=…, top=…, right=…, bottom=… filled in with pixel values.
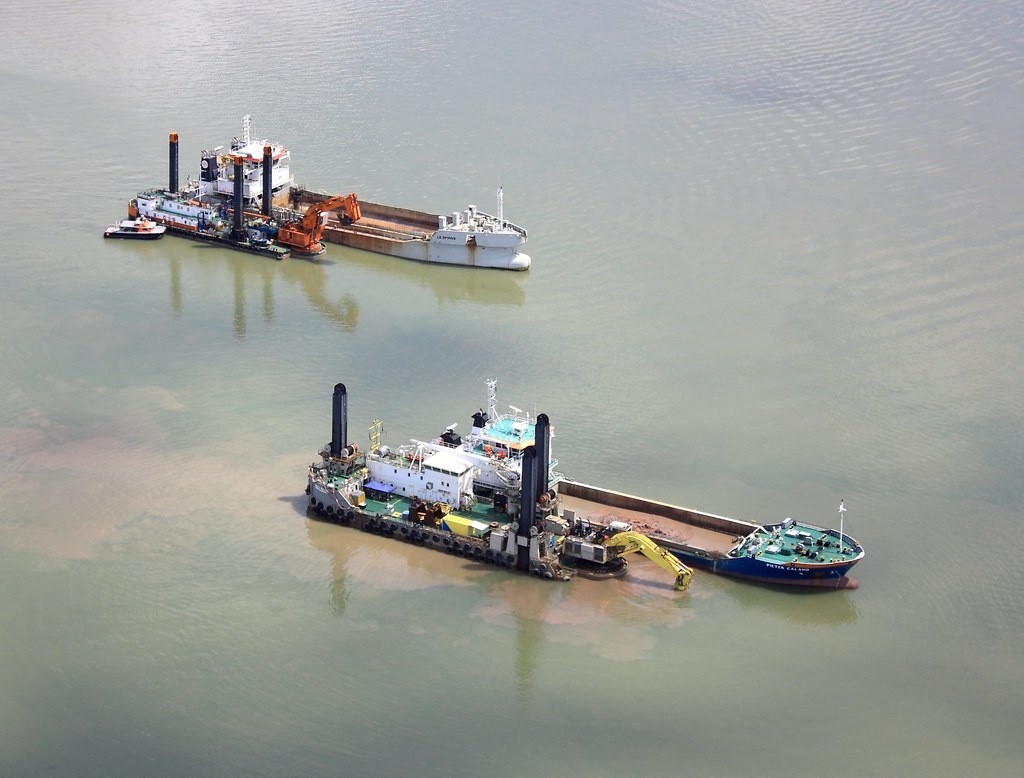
left=302, top=380, right=869, bottom=592
left=127, top=114, right=534, bottom=271
left=102, top=214, right=168, bottom=241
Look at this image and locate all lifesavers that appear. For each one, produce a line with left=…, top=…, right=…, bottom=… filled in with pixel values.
left=311, top=496, right=354, bottom=527
left=231, top=241, right=255, bottom=252
left=168, top=226, right=210, bottom=240
left=532, top=564, right=553, bottom=580
left=433, top=535, right=514, bottom=569
left=362, top=517, right=430, bottom=546
